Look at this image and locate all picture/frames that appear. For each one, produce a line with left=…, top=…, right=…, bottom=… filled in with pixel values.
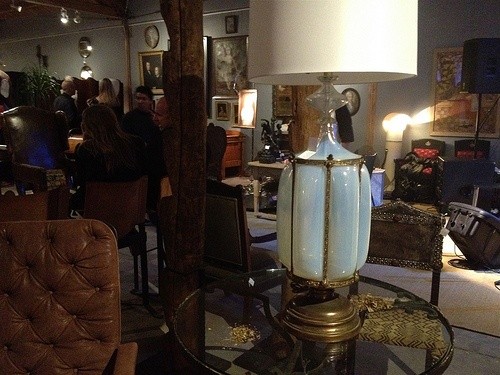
left=210, top=35, right=248, bottom=100
left=431, top=46, right=500, bottom=139
left=216, top=101, right=231, bottom=120
left=225, top=15, right=237, bottom=34
left=139, top=50, right=164, bottom=95
left=232, top=103, right=240, bottom=127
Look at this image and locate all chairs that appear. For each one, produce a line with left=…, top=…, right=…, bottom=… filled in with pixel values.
left=0, top=105, right=490, bottom=375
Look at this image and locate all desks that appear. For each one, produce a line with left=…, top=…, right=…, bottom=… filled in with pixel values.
left=247, top=161, right=287, bottom=221
left=173, top=271, right=455, bottom=375
left=221, top=135, right=247, bottom=178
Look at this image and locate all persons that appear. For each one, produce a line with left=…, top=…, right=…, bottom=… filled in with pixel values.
left=55, top=78, right=222, bottom=221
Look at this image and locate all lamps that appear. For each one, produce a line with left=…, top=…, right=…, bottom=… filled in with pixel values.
left=10, top=0, right=82, bottom=25
left=461, top=38, right=500, bottom=159
left=250, top=0, right=418, bottom=343
